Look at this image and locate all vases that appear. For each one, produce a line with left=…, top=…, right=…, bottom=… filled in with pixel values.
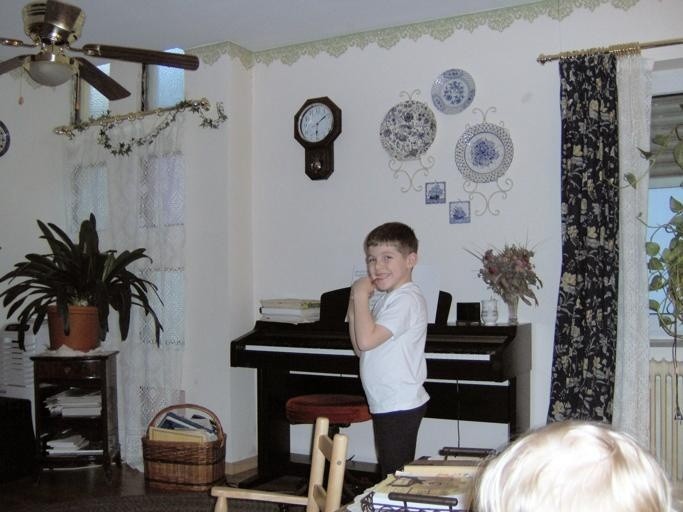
left=507, top=298, right=520, bottom=325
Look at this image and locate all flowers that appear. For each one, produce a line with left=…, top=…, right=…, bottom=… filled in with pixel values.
left=460, top=225, right=544, bottom=313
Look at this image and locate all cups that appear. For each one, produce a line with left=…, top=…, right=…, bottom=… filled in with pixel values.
left=481, top=300, right=498, bottom=325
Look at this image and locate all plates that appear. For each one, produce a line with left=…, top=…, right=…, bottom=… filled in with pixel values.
left=380, top=99, right=437, bottom=160
left=454, top=122, right=514, bottom=184
left=430, top=68, right=475, bottom=115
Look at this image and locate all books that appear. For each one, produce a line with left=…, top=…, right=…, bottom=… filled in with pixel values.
left=258, top=298, right=321, bottom=325
left=46, top=430, right=103, bottom=454
left=57, top=395, right=102, bottom=417
left=371, top=457, right=484, bottom=512
left=149, top=410, right=219, bottom=443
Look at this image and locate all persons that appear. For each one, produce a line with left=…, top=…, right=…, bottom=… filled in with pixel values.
left=469, top=418, right=673, bottom=512
left=344, top=220, right=433, bottom=486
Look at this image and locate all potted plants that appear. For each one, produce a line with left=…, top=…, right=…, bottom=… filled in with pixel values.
left=0, top=213, right=165, bottom=352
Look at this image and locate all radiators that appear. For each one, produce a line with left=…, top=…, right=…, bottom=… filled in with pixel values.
left=647, top=357, right=683, bottom=512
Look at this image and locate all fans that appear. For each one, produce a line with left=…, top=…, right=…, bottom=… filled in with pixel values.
left=0, top=0, right=199, bottom=102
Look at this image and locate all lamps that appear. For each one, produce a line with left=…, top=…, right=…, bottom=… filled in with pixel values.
left=21, top=43, right=80, bottom=87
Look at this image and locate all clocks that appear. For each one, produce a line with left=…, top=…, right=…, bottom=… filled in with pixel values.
left=0, top=119, right=10, bottom=157
left=294, top=95, right=342, bottom=181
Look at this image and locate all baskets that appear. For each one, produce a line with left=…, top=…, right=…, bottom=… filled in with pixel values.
left=141, top=404, right=226, bottom=495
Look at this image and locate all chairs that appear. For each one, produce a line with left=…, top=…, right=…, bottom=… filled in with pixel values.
left=211, top=416, right=349, bottom=512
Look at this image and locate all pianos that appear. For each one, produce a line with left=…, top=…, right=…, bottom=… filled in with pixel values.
left=231, top=321, right=531, bottom=481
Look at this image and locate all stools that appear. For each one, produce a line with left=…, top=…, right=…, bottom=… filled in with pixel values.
left=278, top=393, right=373, bottom=512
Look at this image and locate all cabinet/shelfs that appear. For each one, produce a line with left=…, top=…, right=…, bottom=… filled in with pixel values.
left=28, top=348, right=123, bottom=481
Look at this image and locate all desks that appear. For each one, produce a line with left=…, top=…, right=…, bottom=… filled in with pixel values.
left=334, top=447, right=499, bottom=512
